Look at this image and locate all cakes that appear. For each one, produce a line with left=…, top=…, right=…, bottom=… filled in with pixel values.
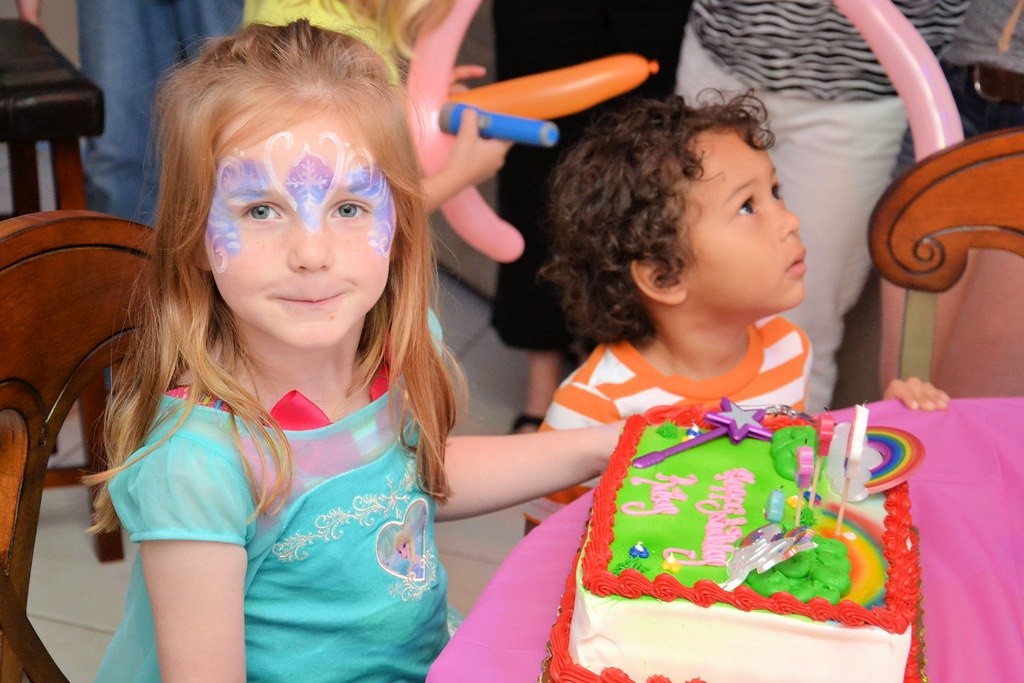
left=551, top=403, right=921, bottom=683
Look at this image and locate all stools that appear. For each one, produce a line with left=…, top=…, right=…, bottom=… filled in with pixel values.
left=0, top=15, right=126, bottom=561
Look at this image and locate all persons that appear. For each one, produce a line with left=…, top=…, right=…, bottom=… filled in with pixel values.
left=673, top=0, right=1024, bottom=411
left=76, top=20, right=628, bottom=683
left=240, top=0, right=513, bottom=216
left=488, top=0, right=694, bottom=436
left=524, top=89, right=951, bottom=538
left=15, top=0, right=246, bottom=390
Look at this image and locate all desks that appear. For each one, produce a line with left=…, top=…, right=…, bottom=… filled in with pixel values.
left=426, top=397, right=1024, bottom=683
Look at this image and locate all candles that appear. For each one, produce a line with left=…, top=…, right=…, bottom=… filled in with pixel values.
left=847, top=400, right=869, bottom=482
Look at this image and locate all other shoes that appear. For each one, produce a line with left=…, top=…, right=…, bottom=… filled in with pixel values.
left=509, top=410, right=543, bottom=436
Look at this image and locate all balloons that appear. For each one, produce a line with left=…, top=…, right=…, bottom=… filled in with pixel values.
left=406, top=0, right=660, bottom=263
left=828, top=0, right=965, bottom=167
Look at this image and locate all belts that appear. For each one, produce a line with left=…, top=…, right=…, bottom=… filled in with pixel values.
left=938, top=57, right=1024, bottom=106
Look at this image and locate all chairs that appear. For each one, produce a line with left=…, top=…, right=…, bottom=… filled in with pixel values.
left=867, top=127, right=1024, bottom=383
left=0, top=209, right=214, bottom=683
